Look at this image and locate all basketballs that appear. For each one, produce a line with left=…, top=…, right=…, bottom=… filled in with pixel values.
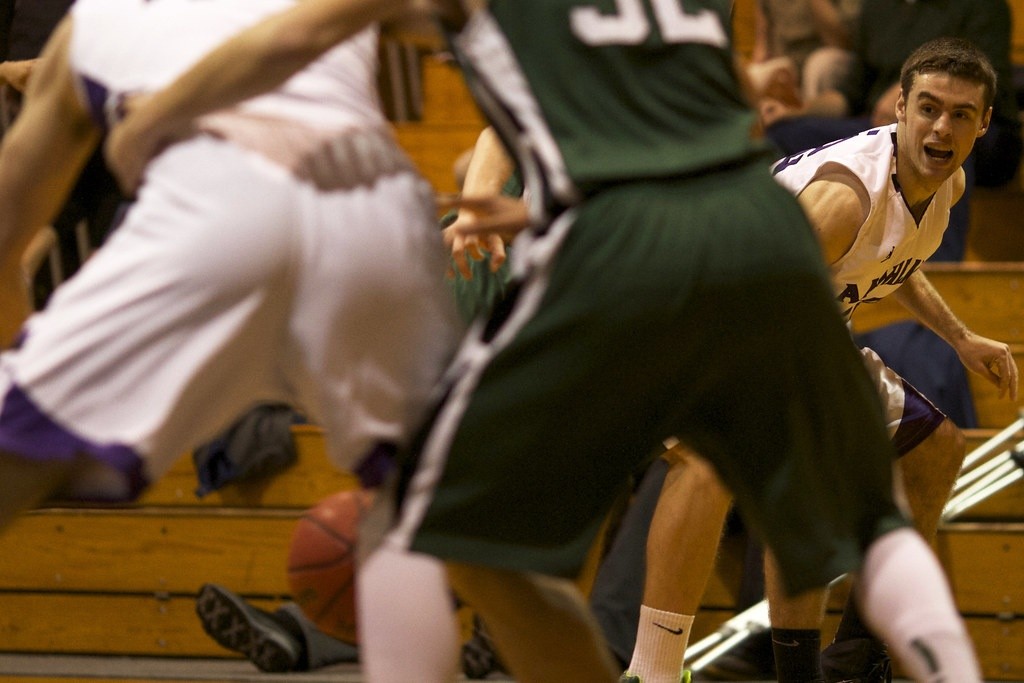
left=284, top=488, right=376, bottom=641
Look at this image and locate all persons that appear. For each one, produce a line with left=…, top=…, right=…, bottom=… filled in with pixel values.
left=0, top=0, right=1024, bottom=682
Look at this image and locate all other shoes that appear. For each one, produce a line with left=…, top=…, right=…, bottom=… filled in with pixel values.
left=462, top=618, right=494, bottom=678
left=196, top=583, right=302, bottom=672
